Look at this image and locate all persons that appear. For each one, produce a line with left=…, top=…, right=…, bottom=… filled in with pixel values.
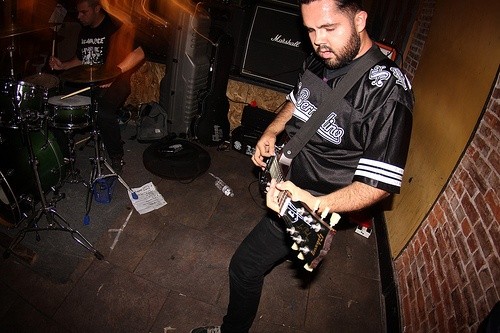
left=48, top=0, right=146, bottom=174
left=188, top=0, right=417, bottom=333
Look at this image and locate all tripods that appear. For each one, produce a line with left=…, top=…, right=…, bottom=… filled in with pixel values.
left=0, top=35, right=139, bottom=261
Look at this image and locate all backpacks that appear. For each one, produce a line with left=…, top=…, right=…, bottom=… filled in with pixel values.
left=136, top=102, right=169, bottom=141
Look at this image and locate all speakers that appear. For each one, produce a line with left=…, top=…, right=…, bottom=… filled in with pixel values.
left=240, top=4, right=316, bottom=90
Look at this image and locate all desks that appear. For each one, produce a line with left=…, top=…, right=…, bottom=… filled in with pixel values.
left=226, top=79, right=289, bottom=137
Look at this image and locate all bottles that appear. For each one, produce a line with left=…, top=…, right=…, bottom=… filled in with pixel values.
left=214, top=179, right=234, bottom=197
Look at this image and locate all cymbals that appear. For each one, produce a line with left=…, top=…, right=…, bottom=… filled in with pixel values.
left=61, top=64, right=121, bottom=82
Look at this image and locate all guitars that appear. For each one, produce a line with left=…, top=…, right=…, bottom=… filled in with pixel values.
left=259, top=140, right=341, bottom=273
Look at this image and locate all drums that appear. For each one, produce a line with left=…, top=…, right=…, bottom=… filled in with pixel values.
left=48, top=95, right=92, bottom=129
left=0, top=125, right=63, bottom=228
left=0, top=79, right=48, bottom=130
left=0, top=22, right=63, bottom=39
left=24, top=73, right=60, bottom=99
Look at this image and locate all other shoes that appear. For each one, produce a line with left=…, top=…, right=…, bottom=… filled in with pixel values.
left=110, top=155, right=123, bottom=172
left=191, top=325, right=222, bottom=333
